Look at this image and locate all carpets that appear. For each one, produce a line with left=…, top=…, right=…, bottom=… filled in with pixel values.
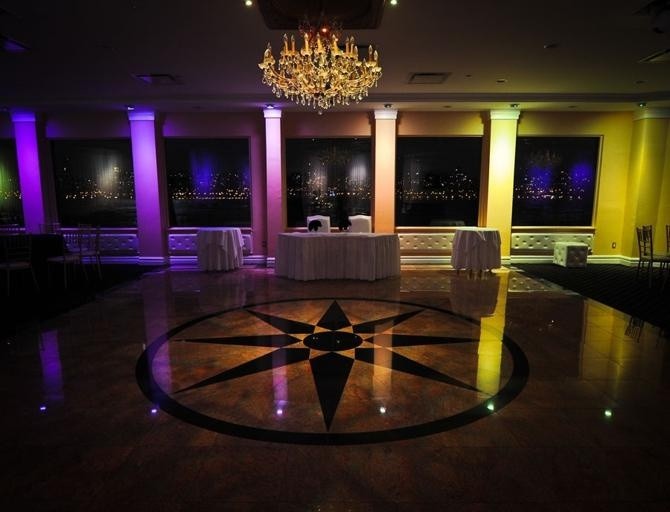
left=513, top=261, right=670, bottom=333
left=0, top=263, right=172, bottom=339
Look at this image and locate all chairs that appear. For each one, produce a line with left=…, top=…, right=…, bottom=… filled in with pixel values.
left=306, top=215, right=330, bottom=233
left=349, top=215, right=371, bottom=233
left=636, top=224, right=670, bottom=289
left=0, top=222, right=101, bottom=296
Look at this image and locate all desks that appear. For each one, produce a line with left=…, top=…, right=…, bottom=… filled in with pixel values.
left=197, top=228, right=244, bottom=272
left=451, top=227, right=501, bottom=275
left=275, top=233, right=400, bottom=282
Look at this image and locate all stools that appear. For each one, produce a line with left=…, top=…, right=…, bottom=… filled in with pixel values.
left=553, top=242, right=588, bottom=268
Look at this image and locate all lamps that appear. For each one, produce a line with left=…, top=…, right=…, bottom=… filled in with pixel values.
left=258, top=9, right=383, bottom=115
